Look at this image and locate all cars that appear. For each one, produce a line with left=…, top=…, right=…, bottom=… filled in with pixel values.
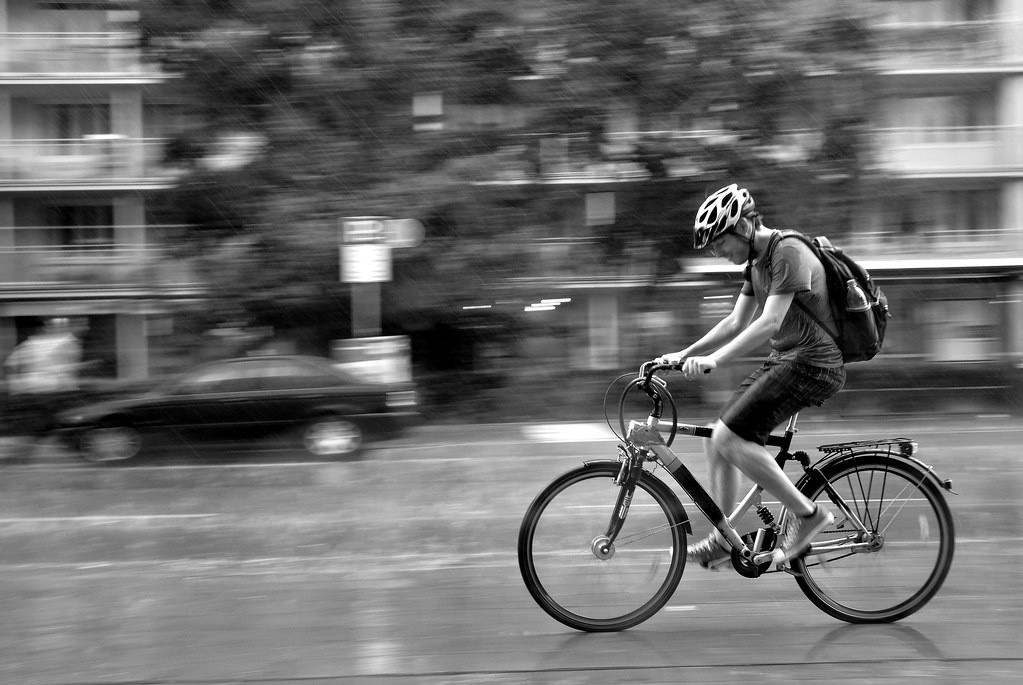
left=55, top=353, right=427, bottom=470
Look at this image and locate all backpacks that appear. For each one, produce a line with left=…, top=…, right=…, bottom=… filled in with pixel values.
left=765, top=229, right=892, bottom=362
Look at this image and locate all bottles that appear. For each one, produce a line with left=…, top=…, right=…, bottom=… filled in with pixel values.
left=847, top=279, right=868, bottom=308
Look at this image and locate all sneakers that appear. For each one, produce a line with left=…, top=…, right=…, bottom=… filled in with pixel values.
left=772, top=503, right=834, bottom=563
left=670, top=530, right=732, bottom=563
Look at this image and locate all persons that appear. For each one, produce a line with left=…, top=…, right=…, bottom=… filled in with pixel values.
left=661, top=184, right=846, bottom=565
left=6, top=319, right=81, bottom=443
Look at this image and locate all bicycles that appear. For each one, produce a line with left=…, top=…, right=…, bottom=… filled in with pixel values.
left=516, top=362, right=959, bottom=633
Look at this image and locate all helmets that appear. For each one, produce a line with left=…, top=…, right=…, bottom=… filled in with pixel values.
left=692, top=183, right=756, bottom=251
